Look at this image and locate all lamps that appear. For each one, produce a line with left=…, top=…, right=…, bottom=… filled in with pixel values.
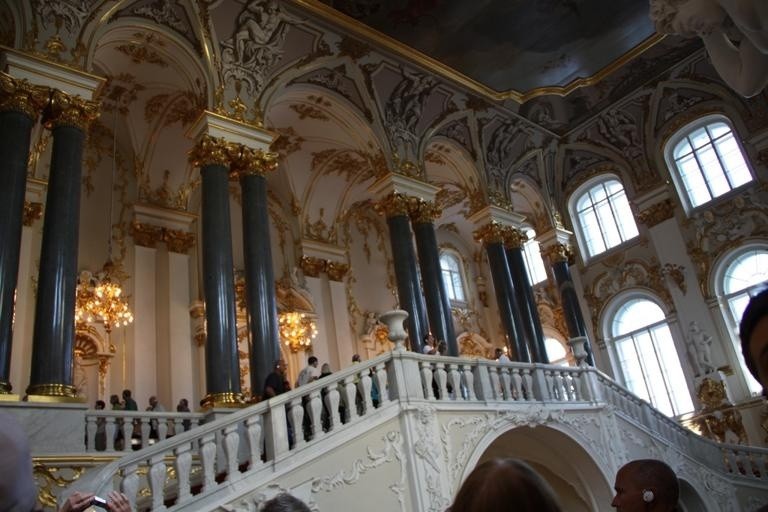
left=73, top=95, right=137, bottom=332
left=276, top=224, right=316, bottom=354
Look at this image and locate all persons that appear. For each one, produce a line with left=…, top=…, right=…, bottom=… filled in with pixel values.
left=612, top=459, right=682, bottom=512
left=58, top=491, right=132, bottom=512
left=263, top=355, right=381, bottom=449
left=445, top=459, right=562, bottom=512
left=685, top=321, right=718, bottom=373
left=93, top=390, right=194, bottom=450
left=260, top=492, right=311, bottom=512
left=0, top=410, right=52, bottom=512
left=739, top=288, right=768, bottom=401
left=423, top=335, right=453, bottom=400
left=495, top=348, right=510, bottom=362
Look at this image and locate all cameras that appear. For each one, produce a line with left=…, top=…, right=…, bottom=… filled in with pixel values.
left=92, top=496, right=108, bottom=507
